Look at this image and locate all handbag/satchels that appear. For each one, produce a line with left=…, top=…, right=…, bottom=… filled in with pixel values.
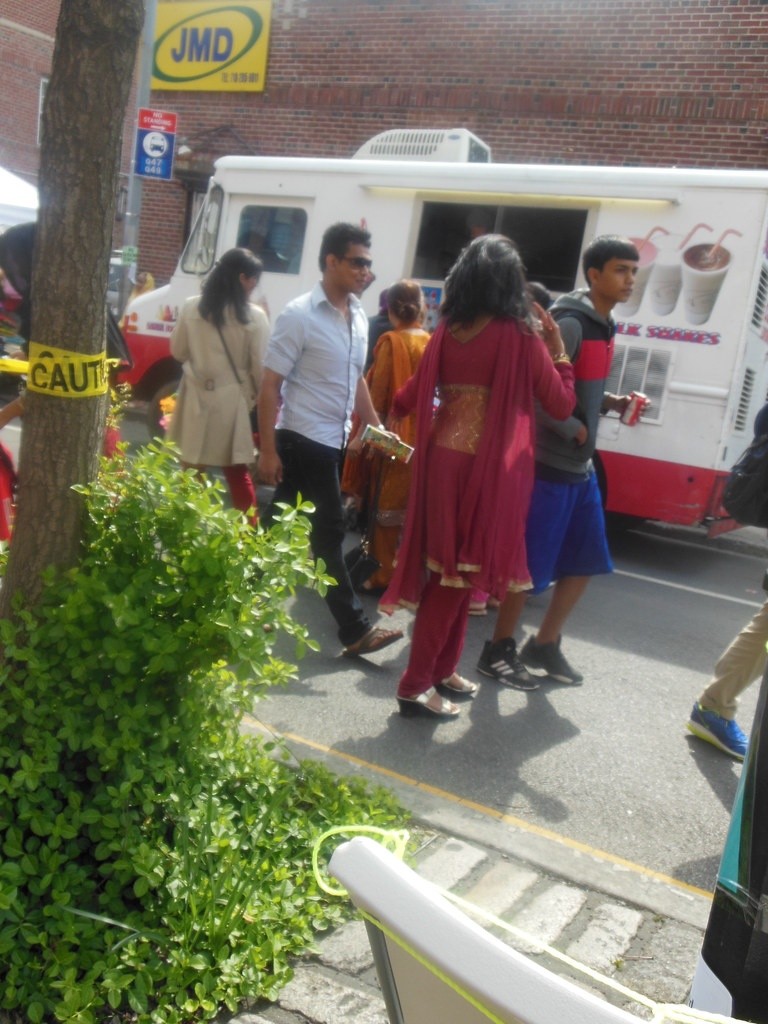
left=239, top=376, right=259, bottom=410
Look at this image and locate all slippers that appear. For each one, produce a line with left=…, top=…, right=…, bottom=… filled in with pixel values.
left=343, top=626, right=404, bottom=658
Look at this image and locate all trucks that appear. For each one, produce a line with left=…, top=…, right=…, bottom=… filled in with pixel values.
left=110, top=129, right=768, bottom=539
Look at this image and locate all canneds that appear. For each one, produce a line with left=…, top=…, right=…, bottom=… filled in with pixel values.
left=619, top=390, right=646, bottom=426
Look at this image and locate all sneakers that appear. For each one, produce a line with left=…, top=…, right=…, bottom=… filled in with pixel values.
left=519, top=633, right=583, bottom=685
left=685, top=702, right=749, bottom=761
left=476, top=636, right=540, bottom=690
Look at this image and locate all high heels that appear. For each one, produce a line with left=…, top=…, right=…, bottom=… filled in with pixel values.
left=395, top=685, right=461, bottom=721
left=433, top=672, right=478, bottom=699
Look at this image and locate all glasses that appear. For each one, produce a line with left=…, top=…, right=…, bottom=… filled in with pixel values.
left=342, top=256, right=372, bottom=270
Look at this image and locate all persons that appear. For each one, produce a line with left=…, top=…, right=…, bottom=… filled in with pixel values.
left=339, top=235, right=652, bottom=692
left=383, top=233, right=579, bottom=722
left=165, top=246, right=272, bottom=528
left=686, top=557, right=768, bottom=763
left=0, top=222, right=132, bottom=543
left=247, top=222, right=404, bottom=660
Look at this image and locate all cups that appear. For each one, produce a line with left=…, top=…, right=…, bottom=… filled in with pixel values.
left=681, top=243, right=732, bottom=325
left=649, top=234, right=690, bottom=315
left=612, top=238, right=657, bottom=316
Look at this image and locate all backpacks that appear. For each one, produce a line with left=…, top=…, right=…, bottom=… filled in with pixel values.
left=722, top=443, right=768, bottom=528
